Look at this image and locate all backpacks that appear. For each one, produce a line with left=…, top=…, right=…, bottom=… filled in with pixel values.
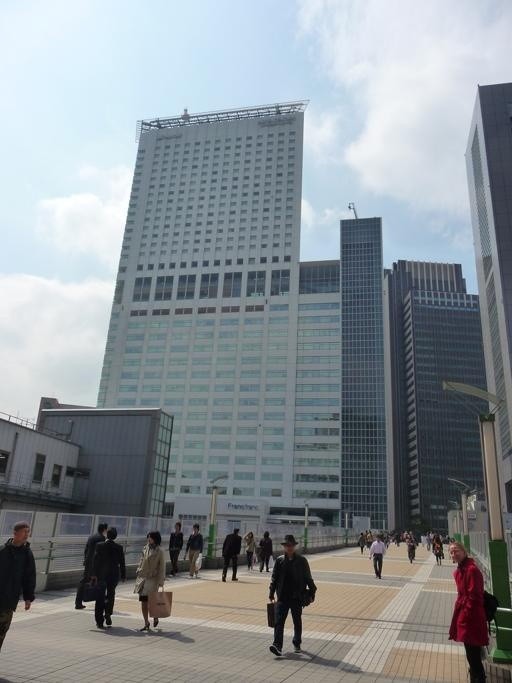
left=484, top=588, right=498, bottom=623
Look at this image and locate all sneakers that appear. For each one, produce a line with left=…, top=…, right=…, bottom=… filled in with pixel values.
left=269, top=644, right=282, bottom=657
left=96, top=613, right=112, bottom=629
left=221, top=574, right=239, bottom=581
left=291, top=638, right=302, bottom=653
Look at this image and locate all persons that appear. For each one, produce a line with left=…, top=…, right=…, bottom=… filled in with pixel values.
left=357, top=529, right=458, bottom=580
left=0, top=522, right=37, bottom=649
left=448, top=542, right=489, bottom=683
left=269, top=535, right=317, bottom=656
left=90, top=527, right=126, bottom=629
left=75, top=524, right=108, bottom=609
left=133, top=531, right=166, bottom=631
left=169, top=522, right=273, bottom=582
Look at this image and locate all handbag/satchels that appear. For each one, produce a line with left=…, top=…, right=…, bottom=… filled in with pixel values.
left=268, top=555, right=275, bottom=568
left=267, top=597, right=276, bottom=627
left=194, top=553, right=203, bottom=572
left=78, top=576, right=106, bottom=602
left=253, top=556, right=256, bottom=564
left=147, top=582, right=173, bottom=618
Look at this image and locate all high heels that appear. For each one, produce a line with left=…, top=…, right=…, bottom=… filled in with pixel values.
left=153, top=618, right=158, bottom=627
left=139, top=622, right=150, bottom=631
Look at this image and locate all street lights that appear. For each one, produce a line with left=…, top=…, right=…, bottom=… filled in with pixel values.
left=205, top=474, right=230, bottom=561
left=302, top=496, right=312, bottom=552
left=437, top=376, right=512, bottom=665
left=341, top=505, right=352, bottom=545
left=445, top=475, right=473, bottom=559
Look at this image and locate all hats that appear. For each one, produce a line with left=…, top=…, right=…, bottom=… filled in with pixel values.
left=279, top=533, right=299, bottom=546
